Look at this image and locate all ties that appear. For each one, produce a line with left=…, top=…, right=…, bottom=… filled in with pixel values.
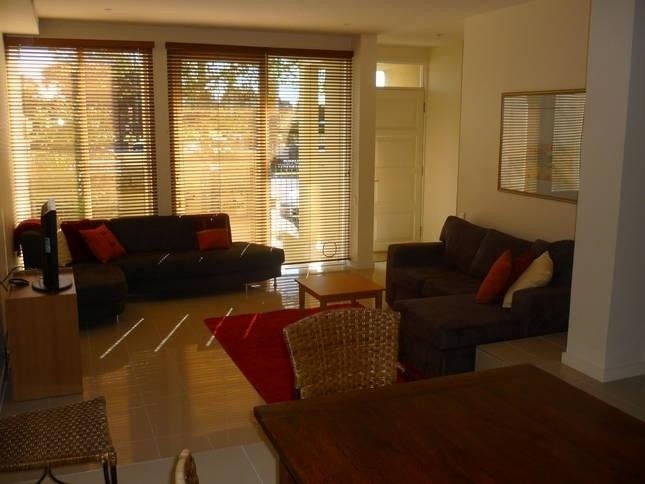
left=30, top=200, right=73, bottom=296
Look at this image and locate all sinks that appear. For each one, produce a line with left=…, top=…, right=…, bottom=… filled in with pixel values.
left=384, top=212, right=575, bottom=379
left=59, top=211, right=285, bottom=330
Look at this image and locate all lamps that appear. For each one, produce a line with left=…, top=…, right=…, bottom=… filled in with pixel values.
left=281, top=308, right=415, bottom=399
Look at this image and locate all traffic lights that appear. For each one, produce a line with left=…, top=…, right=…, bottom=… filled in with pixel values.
left=196, top=226, right=231, bottom=250
left=474, top=245, right=554, bottom=310
left=79, top=223, right=126, bottom=263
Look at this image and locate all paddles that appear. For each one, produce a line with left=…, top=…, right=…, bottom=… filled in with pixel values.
left=204, top=302, right=427, bottom=404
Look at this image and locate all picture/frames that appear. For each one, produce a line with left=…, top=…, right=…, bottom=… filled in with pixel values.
left=253, top=362, right=645, bottom=484
